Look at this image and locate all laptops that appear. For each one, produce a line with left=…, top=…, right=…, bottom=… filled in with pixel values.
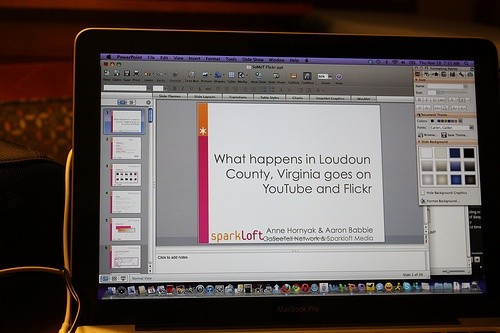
left=68, top=27, right=500, bottom=333
